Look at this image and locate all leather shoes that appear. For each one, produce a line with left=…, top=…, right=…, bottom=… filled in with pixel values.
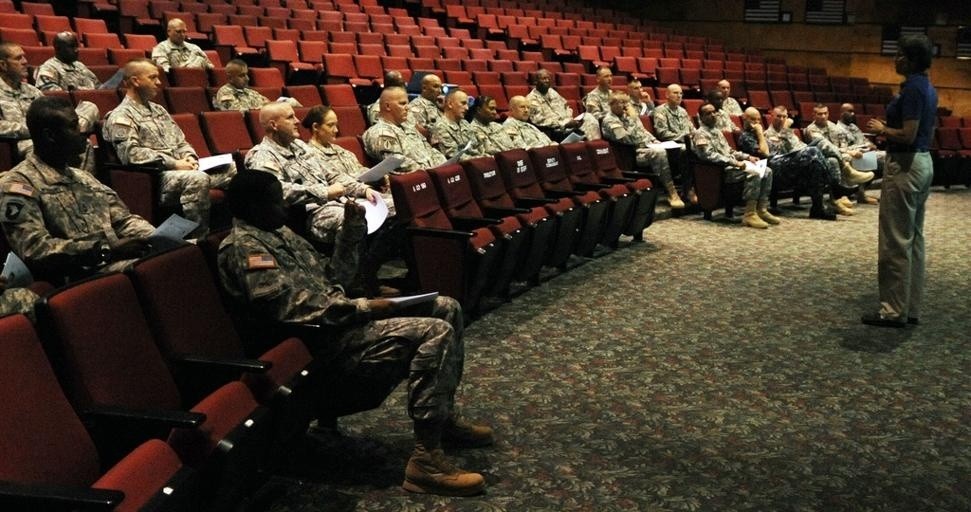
left=860, top=308, right=919, bottom=328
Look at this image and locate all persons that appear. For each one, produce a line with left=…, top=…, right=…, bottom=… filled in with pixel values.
left=1, top=94, right=157, bottom=287
left=216, top=169, right=494, bottom=498
left=0, top=42, right=100, bottom=157
left=212, top=59, right=879, bottom=299
left=861, top=32, right=938, bottom=328
left=100, top=58, right=237, bottom=238
left=152, top=18, right=214, bottom=73
left=34, top=32, right=104, bottom=93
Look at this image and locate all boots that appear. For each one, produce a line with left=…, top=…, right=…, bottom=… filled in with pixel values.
left=400, top=431, right=488, bottom=500
left=809, top=190, right=879, bottom=219
left=740, top=200, right=769, bottom=229
left=757, top=199, right=783, bottom=224
left=663, top=179, right=697, bottom=209
left=842, top=162, right=876, bottom=187
left=438, top=410, right=498, bottom=450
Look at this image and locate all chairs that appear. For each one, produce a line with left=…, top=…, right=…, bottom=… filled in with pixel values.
left=1, top=2, right=310, bottom=510
left=310, top=3, right=971, bottom=342
